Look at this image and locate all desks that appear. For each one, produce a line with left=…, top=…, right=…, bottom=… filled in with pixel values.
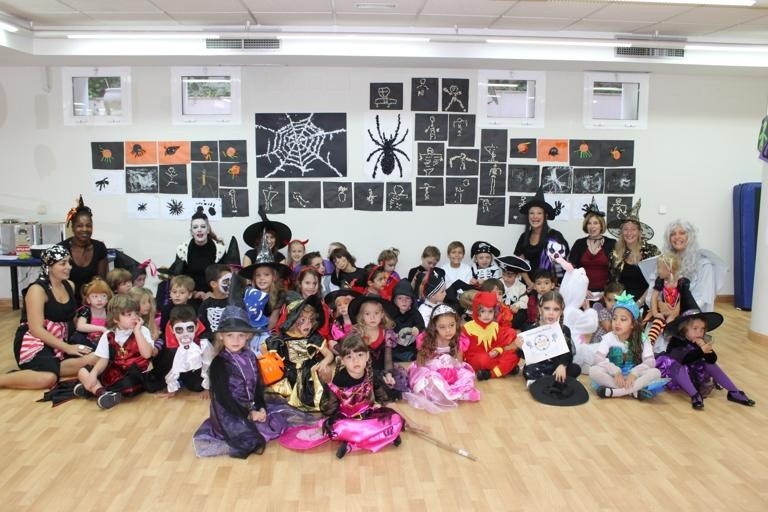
left=0, top=253, right=43, bottom=310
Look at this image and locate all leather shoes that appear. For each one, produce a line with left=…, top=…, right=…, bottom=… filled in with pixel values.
left=632, top=391, right=644, bottom=401
left=692, top=392, right=704, bottom=408
left=727, top=391, right=748, bottom=405
left=597, top=386, right=617, bottom=399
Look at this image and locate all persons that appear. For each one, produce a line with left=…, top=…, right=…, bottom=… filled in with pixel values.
left=146, top=208, right=429, bottom=459
left=406, top=183, right=754, bottom=413
left=0, top=195, right=164, bottom=409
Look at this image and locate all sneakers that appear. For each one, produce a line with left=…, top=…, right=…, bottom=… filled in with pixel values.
left=73, top=384, right=94, bottom=397
left=336, top=440, right=349, bottom=458
left=510, top=366, right=519, bottom=375
left=393, top=435, right=402, bottom=446
left=477, top=369, right=490, bottom=380
left=97, top=391, right=124, bottom=409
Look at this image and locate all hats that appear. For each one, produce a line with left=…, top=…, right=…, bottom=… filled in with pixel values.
left=279, top=422, right=331, bottom=450
left=664, top=283, right=723, bottom=340
left=612, top=298, right=640, bottom=320
left=157, top=260, right=196, bottom=281
left=494, top=256, right=532, bottom=274
left=519, top=185, right=555, bottom=220
left=584, top=196, right=605, bottom=217
left=471, top=241, right=500, bottom=258
left=529, top=374, right=589, bottom=406
left=607, top=198, right=654, bottom=242
left=208, top=206, right=401, bottom=339
left=414, top=268, right=445, bottom=299
